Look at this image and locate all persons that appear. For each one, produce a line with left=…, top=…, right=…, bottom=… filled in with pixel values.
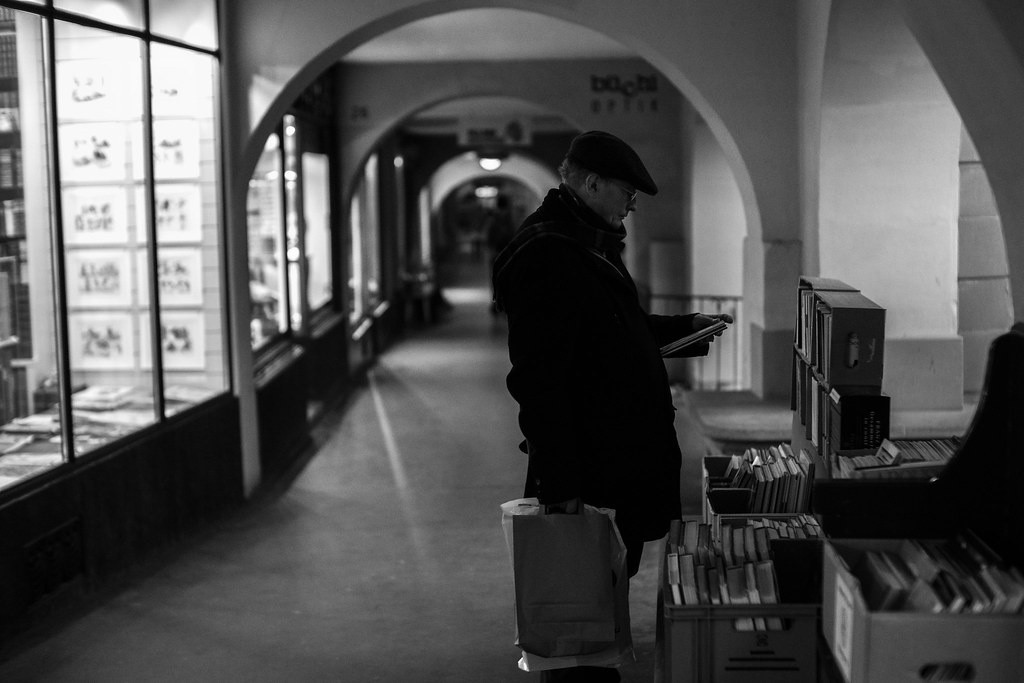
left=493, top=129, right=733, bottom=683
left=483, top=193, right=516, bottom=313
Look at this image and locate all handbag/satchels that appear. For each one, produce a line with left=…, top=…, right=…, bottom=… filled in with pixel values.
left=500, top=490, right=637, bottom=673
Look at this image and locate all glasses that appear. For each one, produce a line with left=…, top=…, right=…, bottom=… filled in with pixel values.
left=604, top=178, right=638, bottom=202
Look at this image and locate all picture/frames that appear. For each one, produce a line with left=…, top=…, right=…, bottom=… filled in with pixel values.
left=139, top=311, right=206, bottom=371
left=134, top=183, right=202, bottom=243
left=60, top=186, right=131, bottom=245
left=65, top=248, right=133, bottom=307
left=130, top=120, right=201, bottom=180
left=58, top=122, right=127, bottom=183
left=136, top=247, right=204, bottom=307
left=69, top=312, right=134, bottom=371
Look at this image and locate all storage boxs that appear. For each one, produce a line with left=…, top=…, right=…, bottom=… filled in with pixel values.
left=653, top=275, right=1024, bottom=683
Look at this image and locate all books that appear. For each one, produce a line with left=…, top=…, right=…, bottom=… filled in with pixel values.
left=658, top=276, right=1024, bottom=683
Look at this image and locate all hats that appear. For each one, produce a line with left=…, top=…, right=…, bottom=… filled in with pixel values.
left=569, top=130, right=659, bottom=196
